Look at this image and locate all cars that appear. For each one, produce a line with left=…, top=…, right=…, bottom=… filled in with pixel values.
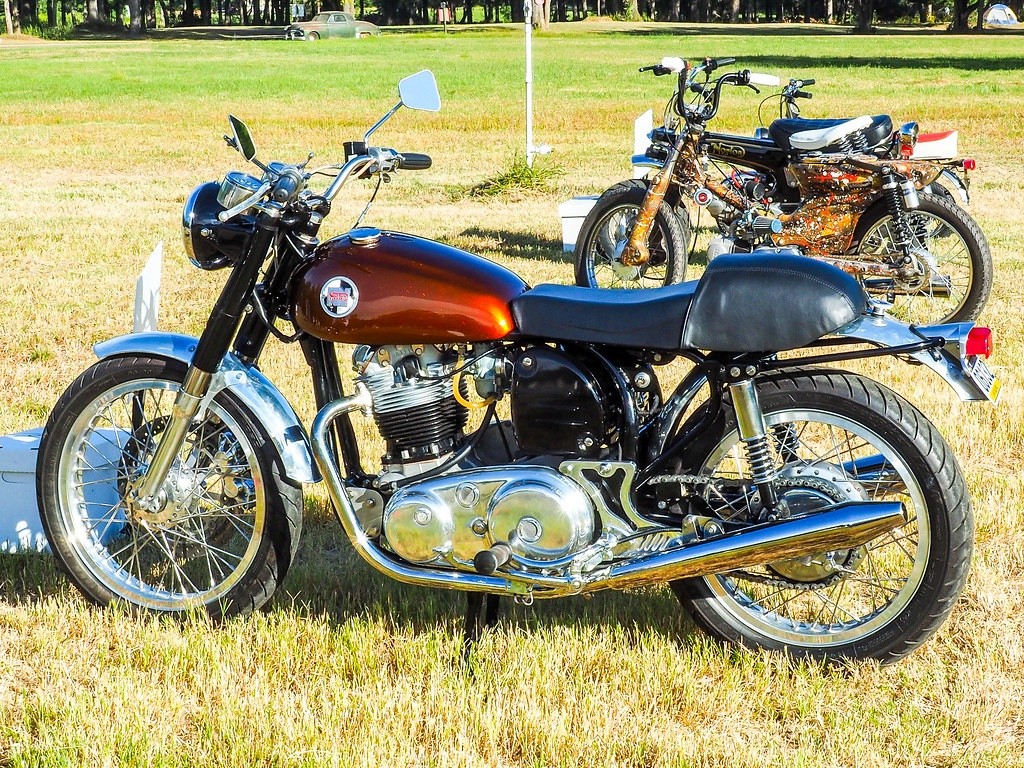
left=283, top=11, right=382, bottom=41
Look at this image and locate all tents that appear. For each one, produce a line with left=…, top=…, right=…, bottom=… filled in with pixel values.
left=983, top=4, right=1017, bottom=24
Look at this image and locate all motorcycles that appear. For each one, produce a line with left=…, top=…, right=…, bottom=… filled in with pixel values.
left=596, top=57, right=895, bottom=263
left=714, top=78, right=976, bottom=240
left=34, top=68, right=1004, bottom=681
left=573, top=57, right=992, bottom=325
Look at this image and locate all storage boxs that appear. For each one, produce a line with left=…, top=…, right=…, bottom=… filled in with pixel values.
left=0, top=428, right=134, bottom=558
left=557, top=196, right=626, bottom=254
